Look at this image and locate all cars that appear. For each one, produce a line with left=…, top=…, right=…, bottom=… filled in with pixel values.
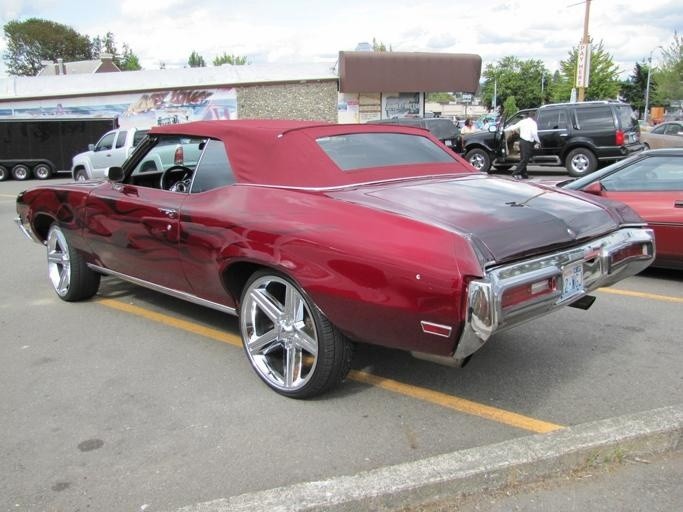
left=518, top=146, right=683, bottom=274
left=640, top=121, right=683, bottom=151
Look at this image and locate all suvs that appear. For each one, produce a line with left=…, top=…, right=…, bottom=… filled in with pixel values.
left=366, top=117, right=466, bottom=158
left=71, top=123, right=153, bottom=182
left=463, top=100, right=652, bottom=178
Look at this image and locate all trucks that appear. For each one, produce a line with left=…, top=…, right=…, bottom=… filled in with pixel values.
left=0, top=116, right=118, bottom=181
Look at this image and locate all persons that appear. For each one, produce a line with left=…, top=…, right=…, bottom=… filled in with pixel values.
left=513, top=109, right=541, bottom=180
left=461, top=119, right=482, bottom=147
left=489, top=104, right=504, bottom=132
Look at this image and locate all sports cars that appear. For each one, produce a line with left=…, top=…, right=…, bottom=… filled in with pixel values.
left=13, top=118, right=658, bottom=400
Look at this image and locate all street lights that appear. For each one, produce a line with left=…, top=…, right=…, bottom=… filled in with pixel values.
left=643, top=45, right=663, bottom=123
